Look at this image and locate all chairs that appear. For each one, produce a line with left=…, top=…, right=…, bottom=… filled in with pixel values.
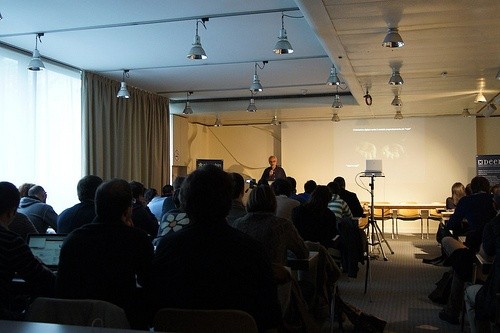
left=28, top=296, right=130, bottom=331
left=360, top=201, right=446, bottom=239
left=154, top=307, right=258, bottom=333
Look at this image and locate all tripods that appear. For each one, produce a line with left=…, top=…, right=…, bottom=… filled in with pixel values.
left=369, top=177, right=395, bottom=260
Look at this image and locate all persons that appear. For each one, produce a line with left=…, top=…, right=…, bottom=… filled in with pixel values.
left=0, top=166, right=368, bottom=333
left=261, top=156, right=286, bottom=181
left=436, top=176, right=500, bottom=333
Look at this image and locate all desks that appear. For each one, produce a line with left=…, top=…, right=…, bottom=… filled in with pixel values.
left=367, top=203, right=446, bottom=234
left=286, top=251, right=319, bottom=271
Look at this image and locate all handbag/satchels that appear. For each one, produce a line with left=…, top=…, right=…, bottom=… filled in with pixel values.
left=428, top=270, right=456, bottom=304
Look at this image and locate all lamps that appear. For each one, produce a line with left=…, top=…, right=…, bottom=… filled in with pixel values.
left=246, top=93, right=257, bottom=112
left=249, top=60, right=268, bottom=92
left=27, top=32, right=45, bottom=72
left=186, top=20, right=208, bottom=60
left=382, top=28, right=405, bottom=119
left=463, top=109, right=471, bottom=119
left=116, top=70, right=130, bottom=98
left=273, top=11, right=304, bottom=55
left=182, top=92, right=195, bottom=115
left=481, top=71, right=500, bottom=118
left=327, top=67, right=343, bottom=124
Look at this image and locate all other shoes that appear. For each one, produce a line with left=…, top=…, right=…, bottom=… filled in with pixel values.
left=439, top=311, right=459, bottom=324
